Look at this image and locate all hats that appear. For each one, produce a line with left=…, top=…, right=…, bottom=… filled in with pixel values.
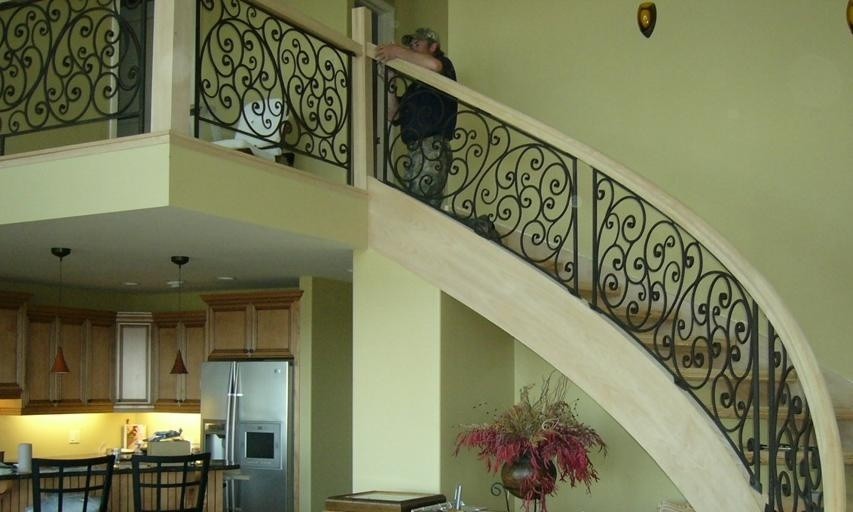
left=401, top=27, right=440, bottom=46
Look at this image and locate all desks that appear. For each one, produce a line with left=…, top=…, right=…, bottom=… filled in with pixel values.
left=325, top=487, right=446, bottom=512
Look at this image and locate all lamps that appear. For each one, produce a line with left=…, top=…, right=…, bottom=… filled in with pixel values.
left=50, top=247, right=73, bottom=373
left=171, top=254, right=190, bottom=373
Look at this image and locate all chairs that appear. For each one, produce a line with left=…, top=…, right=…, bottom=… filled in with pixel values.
left=208, top=99, right=295, bottom=166
left=29, top=455, right=116, bottom=512
left=131, top=453, right=210, bottom=511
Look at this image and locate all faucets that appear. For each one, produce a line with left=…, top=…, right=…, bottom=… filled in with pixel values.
left=151, top=428, right=183, bottom=441
left=454, top=483, right=462, bottom=511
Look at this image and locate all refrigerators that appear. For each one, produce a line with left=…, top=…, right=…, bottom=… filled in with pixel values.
left=198, top=359, right=293, bottom=512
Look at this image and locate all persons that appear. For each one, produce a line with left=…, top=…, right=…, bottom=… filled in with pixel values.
left=371, top=23, right=459, bottom=211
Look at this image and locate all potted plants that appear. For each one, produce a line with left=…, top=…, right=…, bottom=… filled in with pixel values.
left=449, top=370, right=608, bottom=511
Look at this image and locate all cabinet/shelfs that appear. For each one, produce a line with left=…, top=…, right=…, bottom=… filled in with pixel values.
left=85, top=309, right=115, bottom=413
left=24, top=304, right=54, bottom=413
left=55, top=305, right=86, bottom=413
left=114, top=311, right=154, bottom=410
left=199, top=291, right=302, bottom=361
left=0, top=291, right=29, bottom=415
left=154, top=310, right=201, bottom=412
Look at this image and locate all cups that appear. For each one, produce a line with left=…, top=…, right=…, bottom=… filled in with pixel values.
left=107, top=447, right=121, bottom=469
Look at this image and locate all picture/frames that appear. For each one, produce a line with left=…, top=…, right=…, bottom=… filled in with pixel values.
left=121, top=423, right=148, bottom=453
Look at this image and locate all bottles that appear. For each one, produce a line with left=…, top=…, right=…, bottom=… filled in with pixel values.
left=191, top=448, right=200, bottom=466
